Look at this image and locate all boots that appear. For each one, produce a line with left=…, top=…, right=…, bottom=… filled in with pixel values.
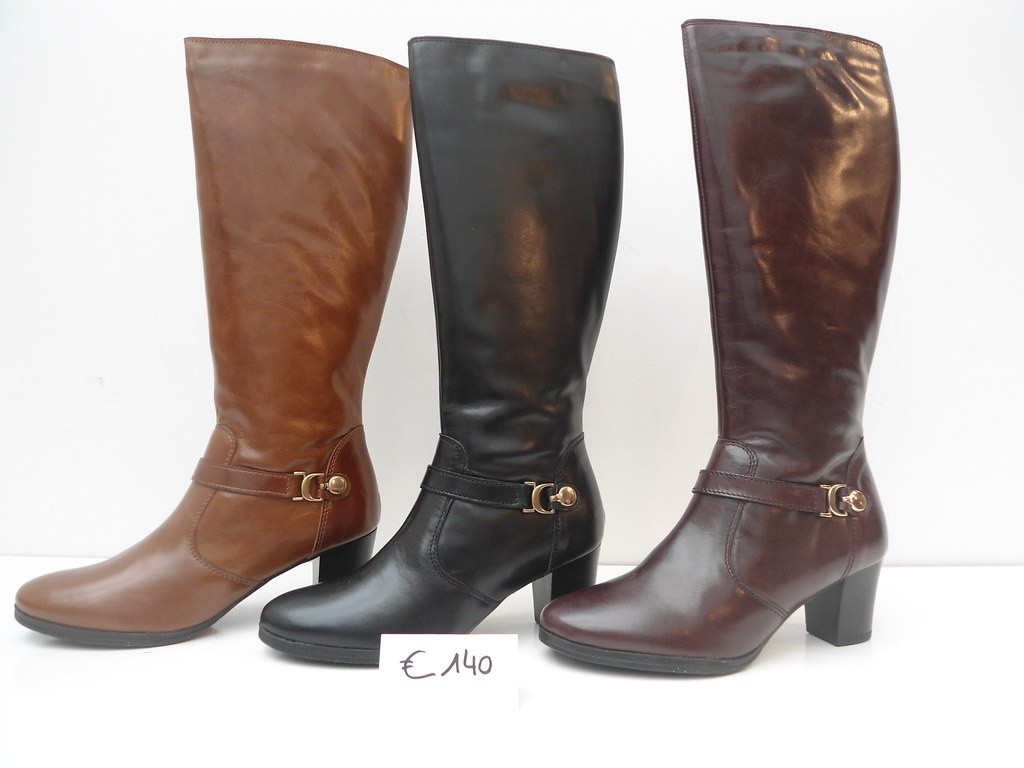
left=15, top=35, right=412, bottom=651
left=260, top=34, right=623, bottom=671
left=539, top=20, right=885, bottom=678
